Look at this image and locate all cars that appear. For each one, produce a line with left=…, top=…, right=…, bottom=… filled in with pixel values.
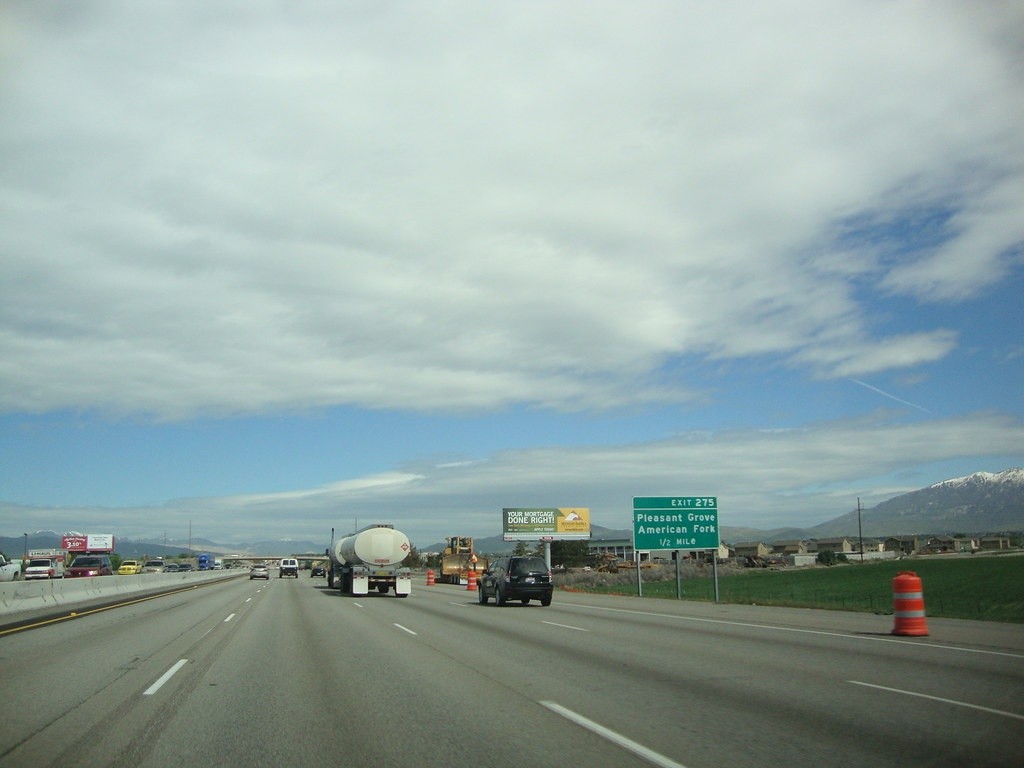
left=225, top=564, right=234, bottom=569
left=179, top=564, right=194, bottom=573
left=763, top=555, right=785, bottom=569
left=310, top=566, right=326, bottom=579
left=213, top=561, right=222, bottom=570
left=250, top=564, right=270, bottom=581
left=235, top=564, right=242, bottom=569
left=167, top=564, right=179, bottom=572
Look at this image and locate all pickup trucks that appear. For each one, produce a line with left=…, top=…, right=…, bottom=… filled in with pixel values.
left=118, top=560, right=143, bottom=576
left=0, top=554, right=22, bottom=582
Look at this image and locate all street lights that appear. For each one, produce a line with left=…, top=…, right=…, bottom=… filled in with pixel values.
left=22, top=533, right=27, bottom=571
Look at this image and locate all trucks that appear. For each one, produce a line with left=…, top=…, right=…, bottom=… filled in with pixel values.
left=198, top=554, right=215, bottom=570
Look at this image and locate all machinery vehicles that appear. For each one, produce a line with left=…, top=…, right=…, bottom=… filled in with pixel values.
left=436, top=535, right=479, bottom=586
left=325, top=524, right=413, bottom=597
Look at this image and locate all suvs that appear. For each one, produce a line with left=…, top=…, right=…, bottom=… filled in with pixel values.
left=477, top=554, right=554, bottom=608
left=25, top=559, right=55, bottom=580
left=63, top=557, right=113, bottom=578
left=278, top=558, right=300, bottom=579
left=141, top=560, right=166, bottom=573
